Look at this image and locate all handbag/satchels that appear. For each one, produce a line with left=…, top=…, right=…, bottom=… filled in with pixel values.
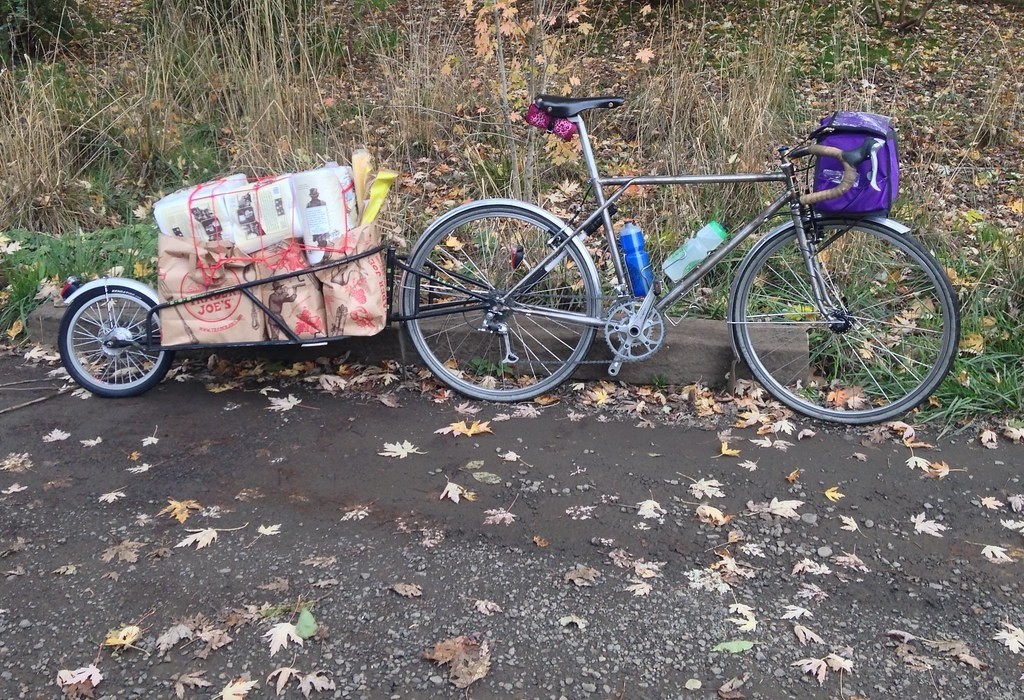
left=813, top=110, right=899, bottom=214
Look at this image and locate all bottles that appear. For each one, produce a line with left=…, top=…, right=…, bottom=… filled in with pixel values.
left=620, top=222, right=654, bottom=297
left=662, top=221, right=728, bottom=283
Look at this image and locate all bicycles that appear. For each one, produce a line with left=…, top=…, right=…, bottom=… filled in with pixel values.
left=57, top=95, right=962, bottom=424
